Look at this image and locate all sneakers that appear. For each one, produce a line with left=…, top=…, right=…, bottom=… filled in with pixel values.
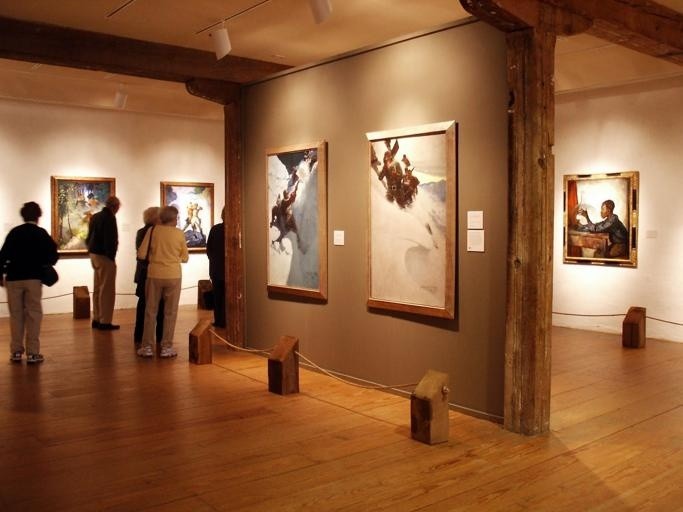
left=159, top=346, right=177, bottom=357
left=27, top=354, right=43, bottom=362
left=10, top=351, right=22, bottom=361
left=137, top=345, right=153, bottom=358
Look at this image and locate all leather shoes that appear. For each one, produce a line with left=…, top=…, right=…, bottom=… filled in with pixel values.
left=99, top=324, right=120, bottom=330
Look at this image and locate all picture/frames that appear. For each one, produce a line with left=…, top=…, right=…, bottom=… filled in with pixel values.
left=563, top=172, right=638, bottom=269
left=265, top=140, right=326, bottom=302
left=366, top=121, right=456, bottom=322
left=50, top=175, right=115, bottom=256
left=160, top=181, right=215, bottom=253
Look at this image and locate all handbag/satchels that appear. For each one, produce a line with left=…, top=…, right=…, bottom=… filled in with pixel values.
left=42, top=261, right=59, bottom=286
left=135, top=225, right=156, bottom=298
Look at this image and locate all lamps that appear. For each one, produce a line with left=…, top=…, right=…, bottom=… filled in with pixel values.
left=212, top=23, right=230, bottom=59
left=306, top=0, right=332, bottom=24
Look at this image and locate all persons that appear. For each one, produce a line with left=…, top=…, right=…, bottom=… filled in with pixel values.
left=133, top=206, right=166, bottom=350
left=278, top=180, right=311, bottom=255
left=182, top=203, right=197, bottom=239
left=377, top=150, right=403, bottom=202
left=0, top=201, right=59, bottom=363
left=84, top=197, right=123, bottom=330
left=270, top=197, right=287, bottom=250
left=136, top=206, right=189, bottom=359
left=580, top=200, right=628, bottom=258
left=206, top=206, right=226, bottom=328
left=191, top=203, right=205, bottom=238
left=86, top=193, right=99, bottom=212
left=76, top=194, right=88, bottom=213
